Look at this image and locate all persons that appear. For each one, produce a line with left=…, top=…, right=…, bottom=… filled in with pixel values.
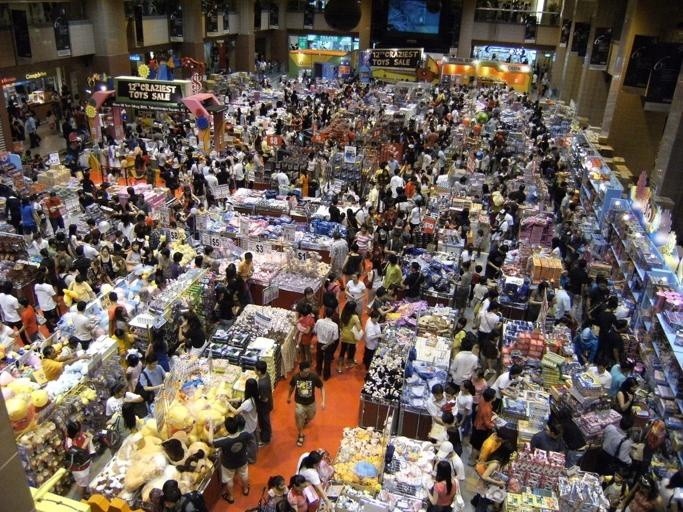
left=53, top=17, right=69, bottom=50
left=202, top=3, right=238, bottom=33
left=304, top=8, right=313, bottom=26
left=158, top=4, right=183, bottom=37
left=1, top=43, right=683, bottom=511
left=271, top=6, right=279, bottom=25
left=475, top=2, right=536, bottom=39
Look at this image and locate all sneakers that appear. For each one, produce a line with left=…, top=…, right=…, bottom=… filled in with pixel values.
left=258, top=441, right=271, bottom=447
left=337, top=368, right=342, bottom=373
left=348, top=363, right=357, bottom=366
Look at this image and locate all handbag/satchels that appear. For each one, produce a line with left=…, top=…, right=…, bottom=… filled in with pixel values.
left=135, top=381, right=155, bottom=405
left=106, top=429, right=122, bottom=449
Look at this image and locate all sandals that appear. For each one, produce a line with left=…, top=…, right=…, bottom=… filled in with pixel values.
left=242, top=484, right=250, bottom=495
left=296, top=434, right=304, bottom=446
left=222, top=492, right=234, bottom=503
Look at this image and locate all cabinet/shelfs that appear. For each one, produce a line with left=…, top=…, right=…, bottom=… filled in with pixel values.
left=0, top=60, right=682, bottom=512
